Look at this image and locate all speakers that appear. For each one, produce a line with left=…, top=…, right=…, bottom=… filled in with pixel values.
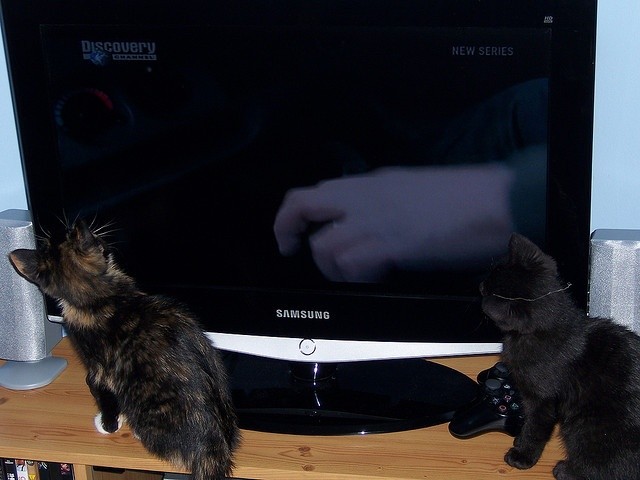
left=588, top=229, right=640, bottom=336
left=1, top=209, right=68, bottom=390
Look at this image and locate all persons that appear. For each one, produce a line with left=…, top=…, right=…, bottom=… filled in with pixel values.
left=274, top=149, right=548, bottom=282
left=59, top=464, right=70, bottom=476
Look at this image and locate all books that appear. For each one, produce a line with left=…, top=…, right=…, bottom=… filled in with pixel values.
left=0, top=457, right=74, bottom=480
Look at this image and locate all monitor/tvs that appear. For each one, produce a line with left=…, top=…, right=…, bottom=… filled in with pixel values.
left=0, top=1, right=596, bottom=434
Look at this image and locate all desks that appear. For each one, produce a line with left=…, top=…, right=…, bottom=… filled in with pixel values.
left=1, top=331, right=565, bottom=479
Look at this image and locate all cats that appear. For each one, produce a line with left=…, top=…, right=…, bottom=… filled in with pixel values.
left=9, top=220, right=242, bottom=480
left=479, top=232, right=640, bottom=479
